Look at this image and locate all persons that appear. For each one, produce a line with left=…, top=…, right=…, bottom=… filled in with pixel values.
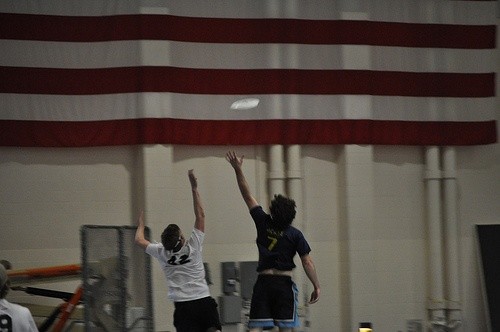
left=225, top=151, right=320, bottom=332
left=134, top=168, right=221, bottom=332
left=0, top=260, right=39, bottom=332
